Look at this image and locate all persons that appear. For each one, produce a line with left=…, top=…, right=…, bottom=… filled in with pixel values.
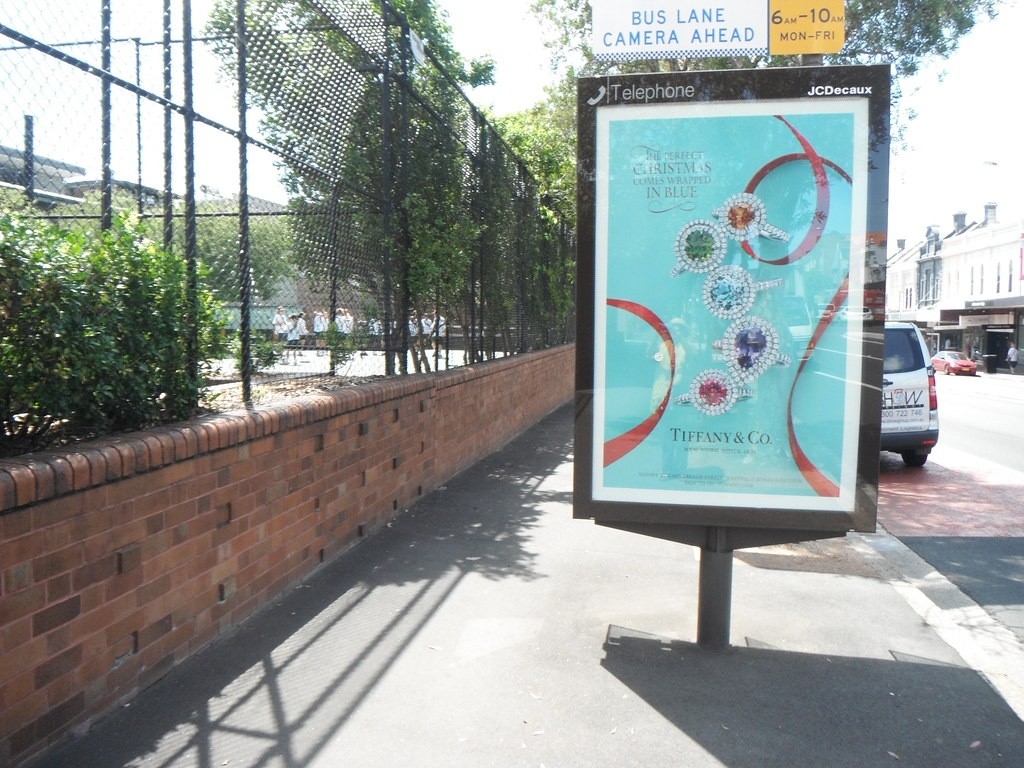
left=1006, top=343, right=1020, bottom=376
left=271, top=304, right=448, bottom=365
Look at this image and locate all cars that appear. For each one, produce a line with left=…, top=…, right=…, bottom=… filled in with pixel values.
left=651, top=293, right=877, bottom=475
left=929, top=349, right=977, bottom=376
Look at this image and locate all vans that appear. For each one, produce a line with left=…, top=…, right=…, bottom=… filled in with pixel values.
left=880, top=322, right=940, bottom=471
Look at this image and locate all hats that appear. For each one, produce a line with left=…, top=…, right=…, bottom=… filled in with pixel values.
left=276, top=306, right=285, bottom=311
